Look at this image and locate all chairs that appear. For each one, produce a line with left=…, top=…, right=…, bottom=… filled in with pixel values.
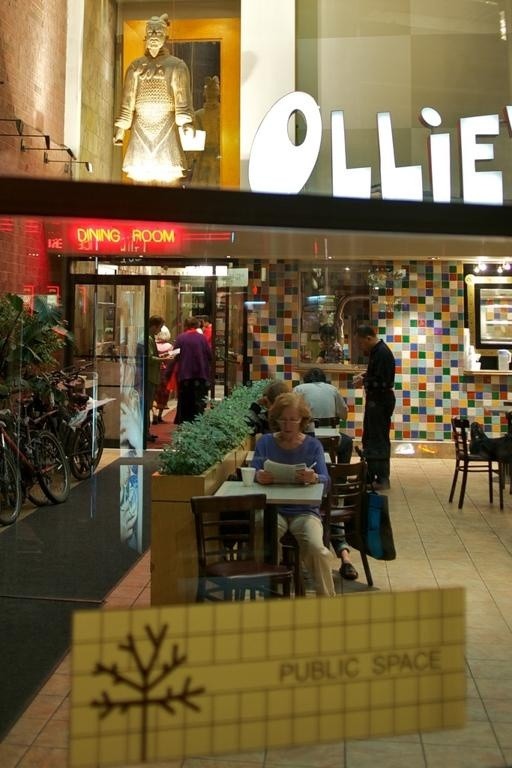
left=190, top=415, right=375, bottom=604
left=448, top=401, right=512, bottom=511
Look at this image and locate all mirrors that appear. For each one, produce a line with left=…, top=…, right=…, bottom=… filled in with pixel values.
left=165, top=34, right=221, bottom=188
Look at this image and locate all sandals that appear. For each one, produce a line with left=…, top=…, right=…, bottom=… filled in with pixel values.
left=339, top=562, right=359, bottom=582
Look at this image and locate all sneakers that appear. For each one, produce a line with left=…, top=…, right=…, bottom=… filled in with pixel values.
left=151, top=415, right=166, bottom=425
left=368, top=478, right=391, bottom=492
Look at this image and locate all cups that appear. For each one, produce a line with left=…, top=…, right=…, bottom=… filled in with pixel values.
left=498, top=350, right=511, bottom=371
left=240, top=467, right=256, bottom=487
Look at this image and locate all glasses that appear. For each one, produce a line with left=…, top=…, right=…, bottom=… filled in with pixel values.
left=274, top=418, right=304, bottom=425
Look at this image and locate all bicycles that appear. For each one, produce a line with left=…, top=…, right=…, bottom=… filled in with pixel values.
left=0, top=353, right=113, bottom=527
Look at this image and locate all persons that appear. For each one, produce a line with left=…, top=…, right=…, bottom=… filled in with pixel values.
left=138, top=310, right=215, bottom=444
left=112, top=14, right=196, bottom=189
left=192, top=74, right=221, bottom=191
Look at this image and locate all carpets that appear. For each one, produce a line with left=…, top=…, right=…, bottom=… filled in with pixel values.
left=146, top=401, right=212, bottom=450
left=0, top=595, right=106, bottom=742
left=0, top=450, right=162, bottom=602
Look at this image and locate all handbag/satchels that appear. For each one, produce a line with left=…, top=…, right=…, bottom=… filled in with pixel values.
left=343, top=494, right=396, bottom=562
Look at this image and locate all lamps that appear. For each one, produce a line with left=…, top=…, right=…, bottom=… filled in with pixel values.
left=20, top=139, right=77, bottom=161
left=0, top=134, right=50, bottom=151
left=0, top=118, right=24, bottom=136
left=44, top=152, right=93, bottom=173
